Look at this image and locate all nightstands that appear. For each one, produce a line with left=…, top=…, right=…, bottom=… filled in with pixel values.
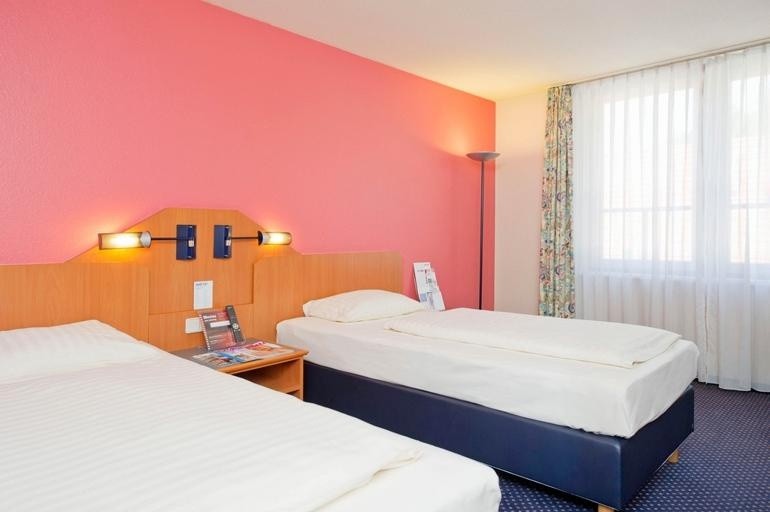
left=169, top=339, right=308, bottom=397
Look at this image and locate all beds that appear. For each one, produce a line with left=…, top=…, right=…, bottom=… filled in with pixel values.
left=276, top=306, right=697, bottom=512
left=1, top=324, right=500, bottom=512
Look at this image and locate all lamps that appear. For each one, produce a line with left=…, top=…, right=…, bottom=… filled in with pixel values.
left=213, top=224, right=294, bottom=259
left=97, top=225, right=198, bottom=262
left=467, top=148, right=501, bottom=309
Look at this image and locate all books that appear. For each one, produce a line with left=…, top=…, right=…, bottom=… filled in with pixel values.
left=413, top=261, right=446, bottom=311
left=193, top=308, right=294, bottom=370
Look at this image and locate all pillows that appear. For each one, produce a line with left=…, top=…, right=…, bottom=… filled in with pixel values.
left=0, top=320, right=164, bottom=380
left=303, top=290, right=423, bottom=322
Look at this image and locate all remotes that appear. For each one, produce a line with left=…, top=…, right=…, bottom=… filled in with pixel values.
left=225, top=304, right=243, bottom=342
